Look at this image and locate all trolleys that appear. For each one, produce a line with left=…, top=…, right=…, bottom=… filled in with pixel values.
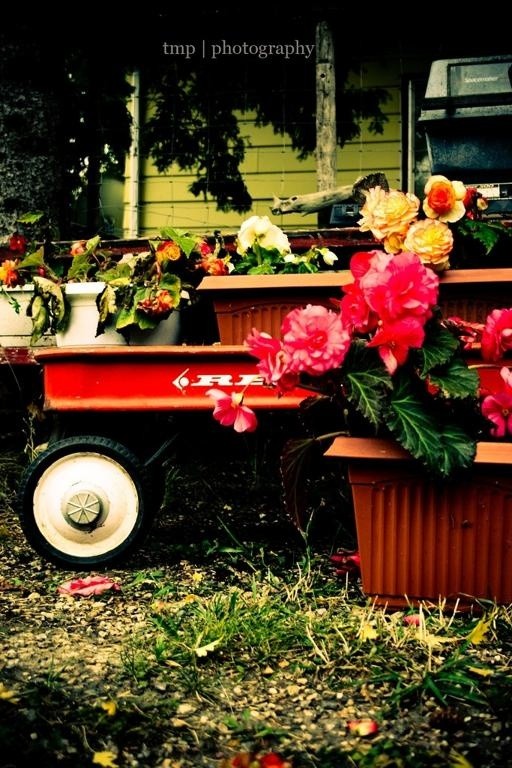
left=12, top=338, right=512, bottom=572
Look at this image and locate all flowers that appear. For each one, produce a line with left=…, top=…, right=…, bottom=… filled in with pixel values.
left=204, top=249, right=512, bottom=477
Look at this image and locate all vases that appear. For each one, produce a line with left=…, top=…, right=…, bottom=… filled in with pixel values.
left=323, top=433, right=510, bottom=613
